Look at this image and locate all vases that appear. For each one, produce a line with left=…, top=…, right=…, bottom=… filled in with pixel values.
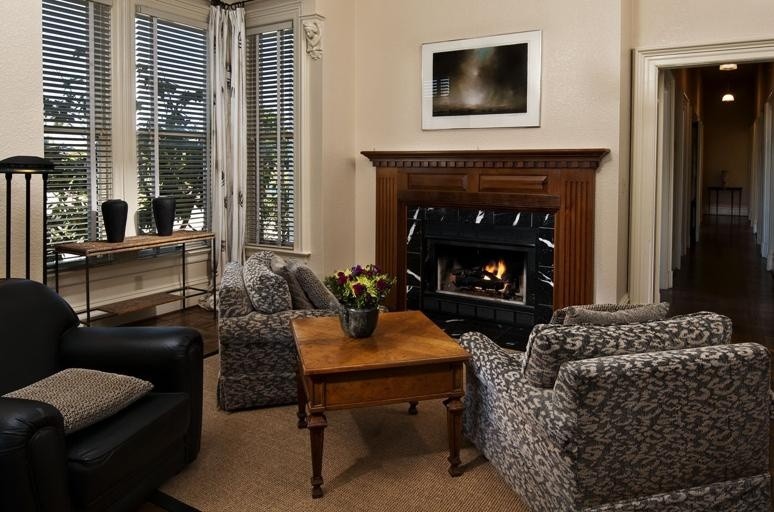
left=152, top=195, right=175, bottom=236
left=102, top=199, right=129, bottom=242
left=338, top=305, right=380, bottom=340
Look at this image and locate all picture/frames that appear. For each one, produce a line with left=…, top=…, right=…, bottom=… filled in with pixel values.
left=421, top=28, right=542, bottom=131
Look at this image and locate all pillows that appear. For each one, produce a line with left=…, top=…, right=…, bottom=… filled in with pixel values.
left=269, top=251, right=313, bottom=313
left=292, top=261, right=341, bottom=311
left=562, top=301, right=672, bottom=326
left=0, top=365, right=151, bottom=439
left=549, top=303, right=652, bottom=327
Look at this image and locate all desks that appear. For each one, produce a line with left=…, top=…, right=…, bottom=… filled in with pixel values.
left=54, top=227, right=219, bottom=329
left=287, top=309, right=474, bottom=502
left=706, top=185, right=743, bottom=221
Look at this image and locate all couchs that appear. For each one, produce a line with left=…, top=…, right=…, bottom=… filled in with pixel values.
left=214, top=261, right=337, bottom=415
left=0, top=277, right=205, bottom=512
left=459, top=311, right=774, bottom=511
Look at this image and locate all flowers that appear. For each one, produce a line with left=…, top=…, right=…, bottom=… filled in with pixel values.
left=324, top=261, right=399, bottom=314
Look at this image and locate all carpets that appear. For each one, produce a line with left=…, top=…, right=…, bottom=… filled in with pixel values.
left=157, top=346, right=526, bottom=511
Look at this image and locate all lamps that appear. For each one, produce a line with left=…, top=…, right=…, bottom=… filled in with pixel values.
left=721, top=94, right=735, bottom=102
left=719, top=64, right=738, bottom=70
